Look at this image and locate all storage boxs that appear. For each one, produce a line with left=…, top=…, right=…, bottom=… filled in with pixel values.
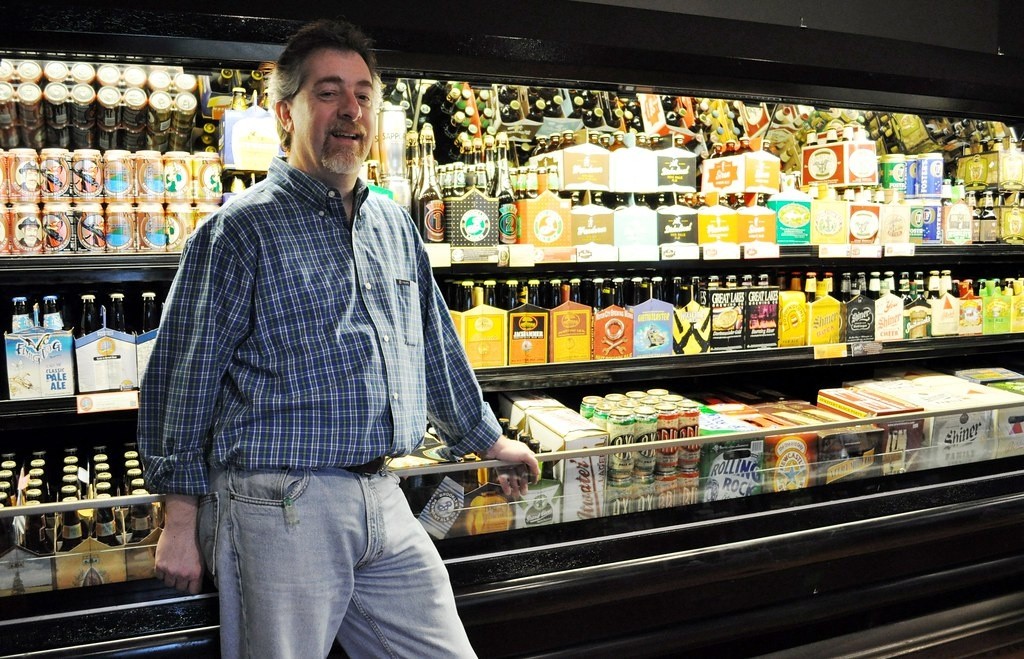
left=0, top=139, right=1024, bottom=603
left=697, top=360, right=1024, bottom=501
left=501, top=393, right=613, bottom=519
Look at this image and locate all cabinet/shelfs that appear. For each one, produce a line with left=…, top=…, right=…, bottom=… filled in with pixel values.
left=0, top=0, right=1024, bottom=659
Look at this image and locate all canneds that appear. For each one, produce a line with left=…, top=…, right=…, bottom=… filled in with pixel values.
left=580, top=389, right=700, bottom=481
left=0, top=146, right=221, bottom=254
left=606, top=468, right=699, bottom=515
left=0, top=60, right=198, bottom=136
left=875, top=152, right=943, bottom=244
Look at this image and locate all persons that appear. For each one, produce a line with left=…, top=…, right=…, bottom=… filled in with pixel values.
left=136, top=21, right=540, bottom=659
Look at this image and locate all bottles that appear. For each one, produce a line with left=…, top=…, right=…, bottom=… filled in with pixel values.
left=42, top=295, right=66, bottom=332
left=111, top=293, right=126, bottom=334
left=205, top=68, right=1024, bottom=312
left=12, top=295, right=33, bottom=334
left=79, top=294, right=99, bottom=337
left=141, top=292, right=159, bottom=334
left=0, top=440, right=152, bottom=582
